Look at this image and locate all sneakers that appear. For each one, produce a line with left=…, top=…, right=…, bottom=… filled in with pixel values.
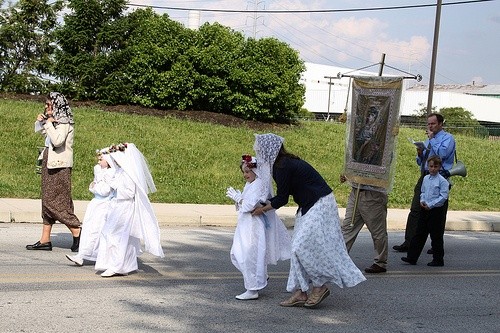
left=365, top=264, right=386, bottom=273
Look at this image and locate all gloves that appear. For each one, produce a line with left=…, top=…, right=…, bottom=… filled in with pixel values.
left=104, top=175, right=117, bottom=190
left=226, top=187, right=242, bottom=204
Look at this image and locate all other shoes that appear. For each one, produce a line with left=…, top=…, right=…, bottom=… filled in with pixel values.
left=267, top=274, right=269, bottom=281
left=401, top=257, right=416, bottom=265
left=393, top=246, right=406, bottom=251
left=101, top=269, right=117, bottom=276
left=427, top=262, right=444, bottom=267
left=427, top=250, right=433, bottom=254
left=236, top=291, right=258, bottom=300
left=66, top=255, right=84, bottom=266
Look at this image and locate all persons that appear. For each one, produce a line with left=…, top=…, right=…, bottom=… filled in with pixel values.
left=226, top=154, right=277, bottom=300
left=26, top=93, right=83, bottom=252
left=392, top=113, right=456, bottom=254
left=250, top=133, right=338, bottom=309
left=339, top=173, right=388, bottom=273
left=401, top=155, right=450, bottom=267
left=66, top=143, right=139, bottom=278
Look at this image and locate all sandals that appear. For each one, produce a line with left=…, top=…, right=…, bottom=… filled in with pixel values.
left=279, top=290, right=308, bottom=307
left=304, top=288, right=330, bottom=307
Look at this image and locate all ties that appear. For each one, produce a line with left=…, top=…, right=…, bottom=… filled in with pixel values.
left=421, top=143, right=431, bottom=176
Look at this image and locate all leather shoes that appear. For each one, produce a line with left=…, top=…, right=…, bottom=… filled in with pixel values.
left=71, top=227, right=82, bottom=252
left=26, top=241, right=52, bottom=250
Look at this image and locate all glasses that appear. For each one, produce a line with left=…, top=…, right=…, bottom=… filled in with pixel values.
left=46, top=103, right=51, bottom=108
